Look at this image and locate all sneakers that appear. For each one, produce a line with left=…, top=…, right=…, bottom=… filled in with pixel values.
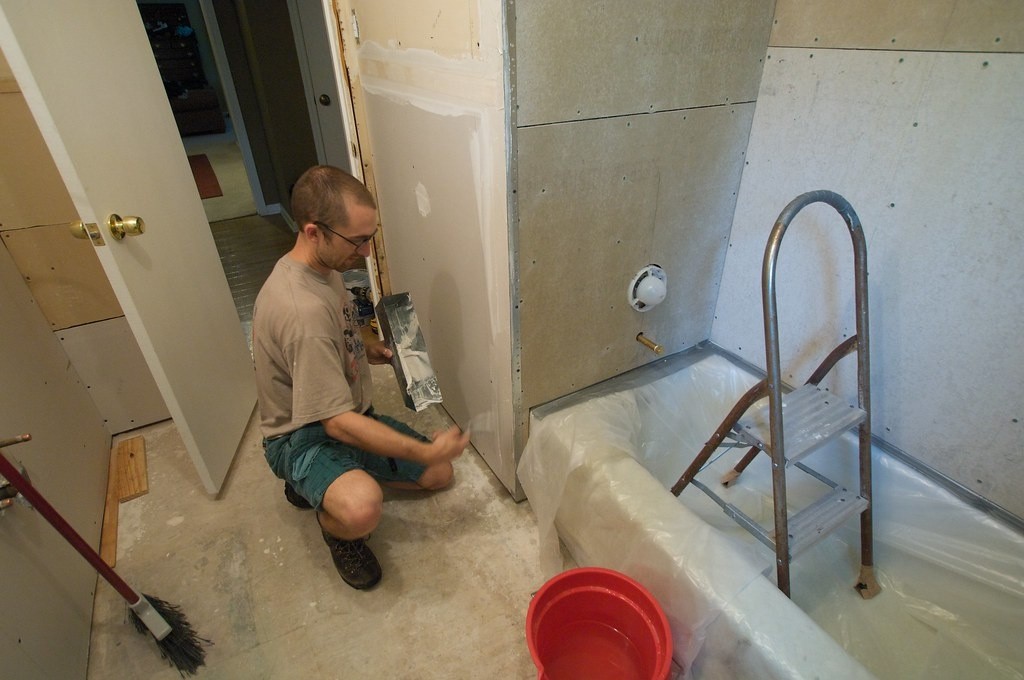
left=316, top=512, right=382, bottom=590
left=285, top=482, right=310, bottom=507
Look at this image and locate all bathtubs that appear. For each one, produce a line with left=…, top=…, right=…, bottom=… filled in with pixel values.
left=528, top=342, right=1024, bottom=676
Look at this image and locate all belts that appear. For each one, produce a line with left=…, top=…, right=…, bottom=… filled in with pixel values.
left=262, top=439, right=268, bottom=447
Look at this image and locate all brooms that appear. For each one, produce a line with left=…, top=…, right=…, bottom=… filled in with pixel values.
left=0, top=455, right=213, bottom=680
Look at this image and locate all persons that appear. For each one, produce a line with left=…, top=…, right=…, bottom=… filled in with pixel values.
left=252, top=165, right=472, bottom=589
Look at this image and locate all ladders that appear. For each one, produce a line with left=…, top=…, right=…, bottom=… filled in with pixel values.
left=671, top=166, right=913, bottom=607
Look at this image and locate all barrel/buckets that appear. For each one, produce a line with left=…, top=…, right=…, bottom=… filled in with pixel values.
left=526, top=568, right=673, bottom=680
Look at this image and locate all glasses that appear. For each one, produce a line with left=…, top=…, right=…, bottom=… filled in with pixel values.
left=313, top=221, right=378, bottom=252
